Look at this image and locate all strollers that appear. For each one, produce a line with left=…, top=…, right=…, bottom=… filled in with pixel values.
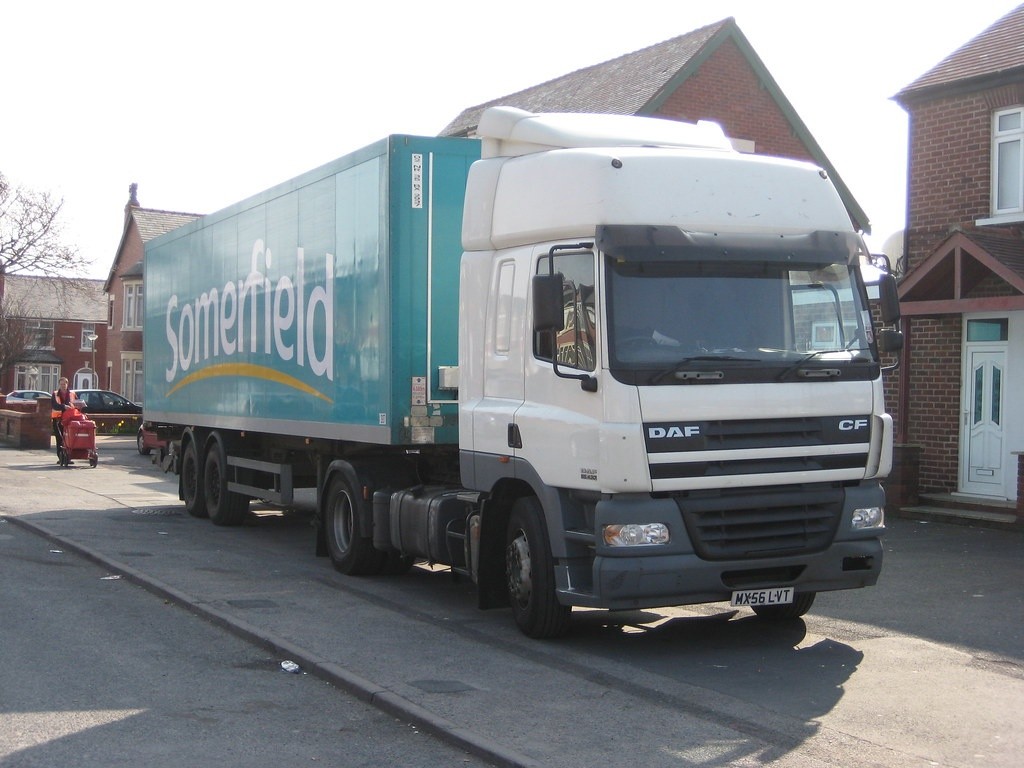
left=56, top=410, right=99, bottom=468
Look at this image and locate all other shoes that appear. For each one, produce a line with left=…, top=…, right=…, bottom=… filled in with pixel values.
left=68, top=461, right=74, bottom=464
left=57, top=461, right=60, bottom=463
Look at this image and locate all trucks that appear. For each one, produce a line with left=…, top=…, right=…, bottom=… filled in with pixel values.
left=144, top=103, right=905, bottom=644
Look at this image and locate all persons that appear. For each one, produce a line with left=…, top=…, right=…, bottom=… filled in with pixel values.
left=50, top=377, right=80, bottom=464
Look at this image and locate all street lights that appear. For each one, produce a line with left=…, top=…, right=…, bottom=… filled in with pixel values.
left=87, top=333, right=99, bottom=388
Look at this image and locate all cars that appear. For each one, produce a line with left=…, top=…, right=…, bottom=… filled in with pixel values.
left=137, top=423, right=168, bottom=456
left=52, top=389, right=143, bottom=414
left=6, top=389, right=52, bottom=404
